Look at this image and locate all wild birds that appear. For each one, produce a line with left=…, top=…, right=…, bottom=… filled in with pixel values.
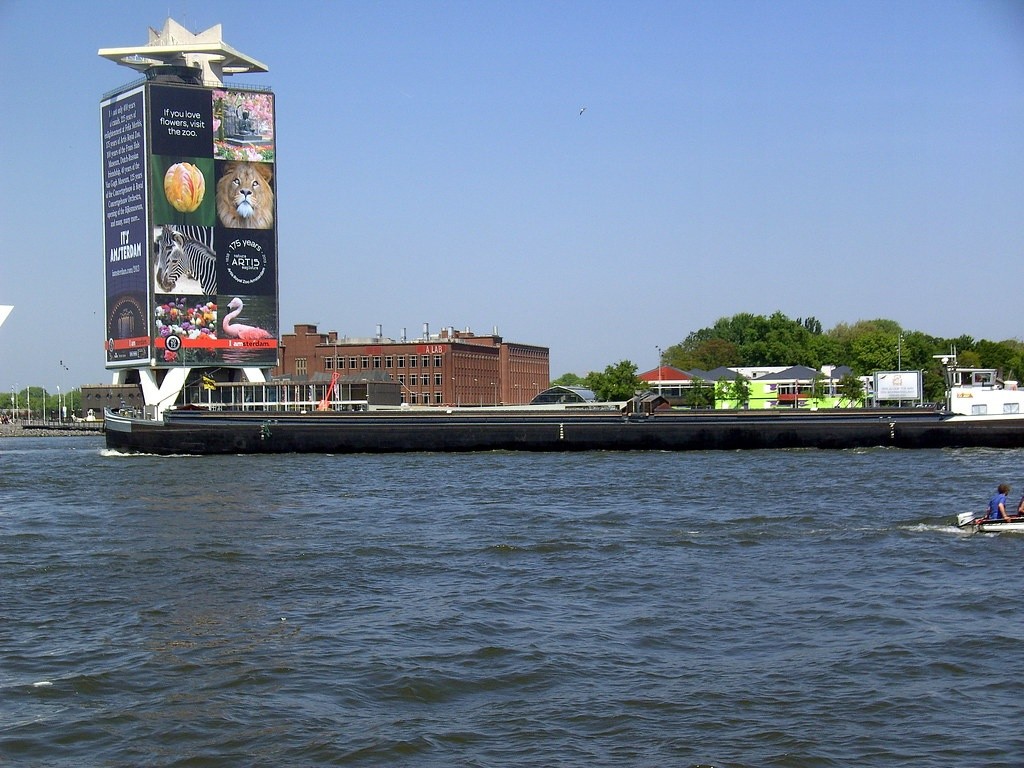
left=578, top=105, right=589, bottom=115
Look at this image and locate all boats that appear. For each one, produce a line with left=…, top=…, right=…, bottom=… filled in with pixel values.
left=104, top=344, right=1023, bottom=456
left=947, top=511, right=1024, bottom=533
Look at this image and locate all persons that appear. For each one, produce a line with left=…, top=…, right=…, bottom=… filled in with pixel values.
left=238, top=111, right=257, bottom=135
left=1017, top=496, right=1024, bottom=517
left=982, top=483, right=1011, bottom=522
left=0, top=414, right=14, bottom=425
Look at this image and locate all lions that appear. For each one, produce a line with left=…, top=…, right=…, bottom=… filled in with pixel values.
left=216, top=161, right=274, bottom=229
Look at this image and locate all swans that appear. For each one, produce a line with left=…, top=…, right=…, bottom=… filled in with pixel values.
left=223, top=297, right=274, bottom=339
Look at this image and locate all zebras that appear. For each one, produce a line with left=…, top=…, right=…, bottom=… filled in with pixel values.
left=154, top=225, right=217, bottom=295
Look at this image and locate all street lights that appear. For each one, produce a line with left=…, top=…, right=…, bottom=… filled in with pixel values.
left=921, top=369, right=924, bottom=406
left=895, top=333, right=905, bottom=408
left=491, top=383, right=496, bottom=407
left=452, top=377, right=460, bottom=407
left=57, top=386, right=61, bottom=424
left=655, top=346, right=664, bottom=395
left=515, top=385, right=521, bottom=406
left=42, top=387, right=45, bottom=424
left=533, top=383, right=539, bottom=394
left=475, top=379, right=482, bottom=407
left=71, top=387, right=74, bottom=422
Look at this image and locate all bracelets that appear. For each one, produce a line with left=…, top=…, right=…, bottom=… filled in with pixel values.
left=1003, top=516, right=1006, bottom=518
